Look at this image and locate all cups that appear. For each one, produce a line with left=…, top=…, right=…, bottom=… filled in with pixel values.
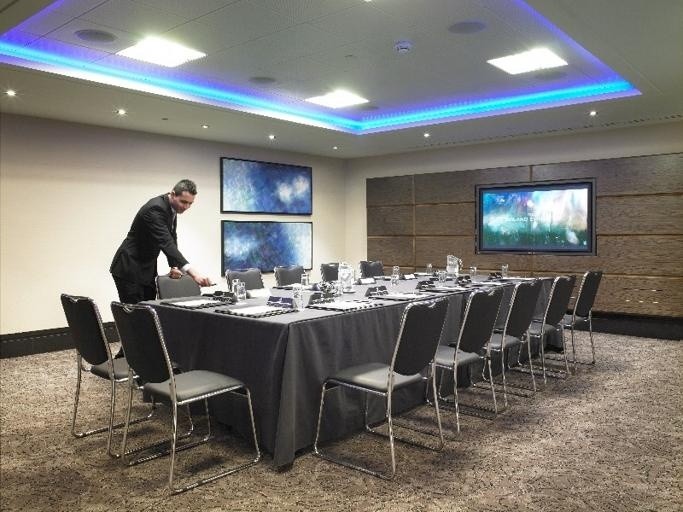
left=230, top=255, right=509, bottom=313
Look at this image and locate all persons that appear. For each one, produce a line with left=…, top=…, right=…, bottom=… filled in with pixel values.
left=110, top=179, right=213, bottom=359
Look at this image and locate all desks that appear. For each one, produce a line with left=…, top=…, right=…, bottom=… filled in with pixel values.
left=138, top=272, right=565, bottom=466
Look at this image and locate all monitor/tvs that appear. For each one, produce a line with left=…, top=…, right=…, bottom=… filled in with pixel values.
left=479, top=183, right=592, bottom=254
left=221, top=220, right=314, bottom=279
left=220, top=156, right=313, bottom=215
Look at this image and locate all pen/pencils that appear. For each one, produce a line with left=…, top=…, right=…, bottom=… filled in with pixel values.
left=272, top=287, right=293, bottom=290
left=199, top=282, right=217, bottom=286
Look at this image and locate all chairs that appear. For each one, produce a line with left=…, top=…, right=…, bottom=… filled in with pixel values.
left=273, top=262, right=309, bottom=290
left=112, top=299, right=262, bottom=494
left=60, top=293, right=196, bottom=449
left=157, top=265, right=204, bottom=301
left=220, top=263, right=269, bottom=294
